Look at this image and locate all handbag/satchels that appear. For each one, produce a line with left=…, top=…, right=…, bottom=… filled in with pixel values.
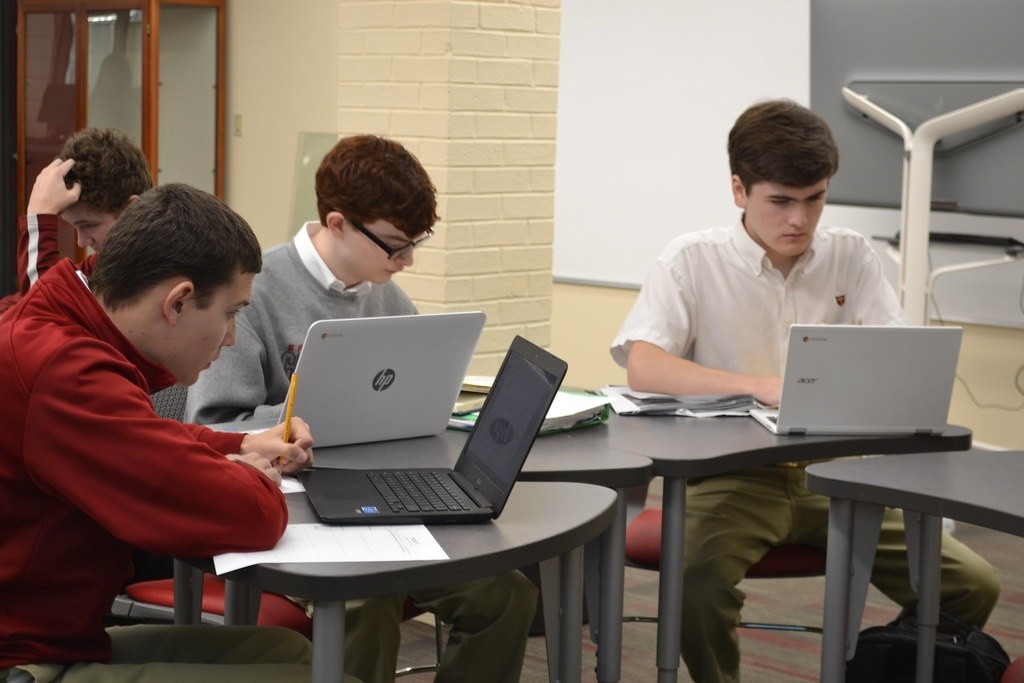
left=847, top=609, right=1010, bottom=682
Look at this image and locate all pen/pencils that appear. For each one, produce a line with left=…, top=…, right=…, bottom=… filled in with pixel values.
left=277, top=374, right=299, bottom=474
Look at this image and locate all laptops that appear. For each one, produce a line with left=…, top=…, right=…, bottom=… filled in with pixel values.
left=206, top=312, right=488, bottom=450
left=298, top=335, right=569, bottom=526
left=751, top=325, right=964, bottom=436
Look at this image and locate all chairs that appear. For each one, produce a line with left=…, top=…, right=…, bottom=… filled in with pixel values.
left=109, top=383, right=304, bottom=633
left=624, top=508, right=825, bottom=635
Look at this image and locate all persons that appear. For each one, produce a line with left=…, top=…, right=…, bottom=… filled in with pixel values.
left=611, top=102, right=1002, bottom=682
left=186, top=137, right=539, bottom=683
left=1, top=125, right=155, bottom=321
left=0, top=185, right=362, bottom=683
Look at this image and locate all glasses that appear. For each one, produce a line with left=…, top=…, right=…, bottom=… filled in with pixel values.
left=351, top=217, right=434, bottom=260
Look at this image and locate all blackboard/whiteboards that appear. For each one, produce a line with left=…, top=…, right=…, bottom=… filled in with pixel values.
left=554, top=1, right=1024, bottom=330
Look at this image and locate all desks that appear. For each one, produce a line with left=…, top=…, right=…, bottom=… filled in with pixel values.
left=802, top=451, right=1024, bottom=683
left=304, top=430, right=654, bottom=683
left=173, top=480, right=618, bottom=683
left=544, top=409, right=973, bottom=683
